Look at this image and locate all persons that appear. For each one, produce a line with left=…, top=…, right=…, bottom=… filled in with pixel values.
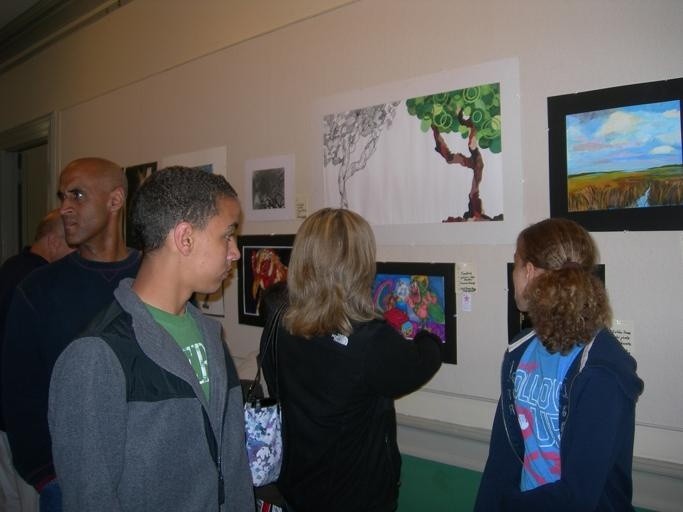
left=260, top=207, right=444, bottom=511
left=474, top=217, right=644, bottom=511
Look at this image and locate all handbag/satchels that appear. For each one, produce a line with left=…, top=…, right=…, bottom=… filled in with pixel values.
left=243, top=299, right=290, bottom=488
